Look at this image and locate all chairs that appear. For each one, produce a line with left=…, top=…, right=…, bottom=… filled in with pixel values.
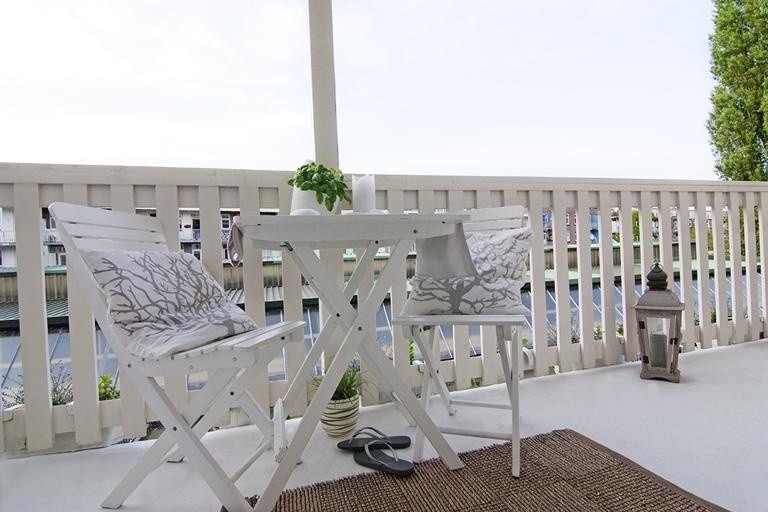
left=390, top=205, right=526, bottom=479
left=50, top=203, right=307, bottom=512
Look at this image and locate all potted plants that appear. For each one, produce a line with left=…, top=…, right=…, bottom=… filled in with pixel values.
left=290, top=162, right=351, bottom=215
left=311, top=367, right=362, bottom=438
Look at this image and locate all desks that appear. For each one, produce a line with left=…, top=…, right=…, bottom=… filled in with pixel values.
left=233, top=210, right=469, bottom=512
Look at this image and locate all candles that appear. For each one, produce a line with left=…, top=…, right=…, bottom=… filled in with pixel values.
left=351, top=174, right=377, bottom=212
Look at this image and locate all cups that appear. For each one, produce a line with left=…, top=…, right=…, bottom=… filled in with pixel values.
left=352, top=172, right=375, bottom=211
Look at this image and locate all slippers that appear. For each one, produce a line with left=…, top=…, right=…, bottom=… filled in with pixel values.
left=337, top=426, right=410, bottom=450
left=354, top=439, right=415, bottom=477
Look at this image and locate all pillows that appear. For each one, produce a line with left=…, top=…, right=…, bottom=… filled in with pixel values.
left=80, top=252, right=255, bottom=362
left=401, top=230, right=529, bottom=317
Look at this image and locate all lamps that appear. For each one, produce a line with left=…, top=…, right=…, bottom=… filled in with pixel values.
left=634, top=263, right=686, bottom=383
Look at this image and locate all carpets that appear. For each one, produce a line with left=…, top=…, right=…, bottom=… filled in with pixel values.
left=221, top=428, right=731, bottom=512
